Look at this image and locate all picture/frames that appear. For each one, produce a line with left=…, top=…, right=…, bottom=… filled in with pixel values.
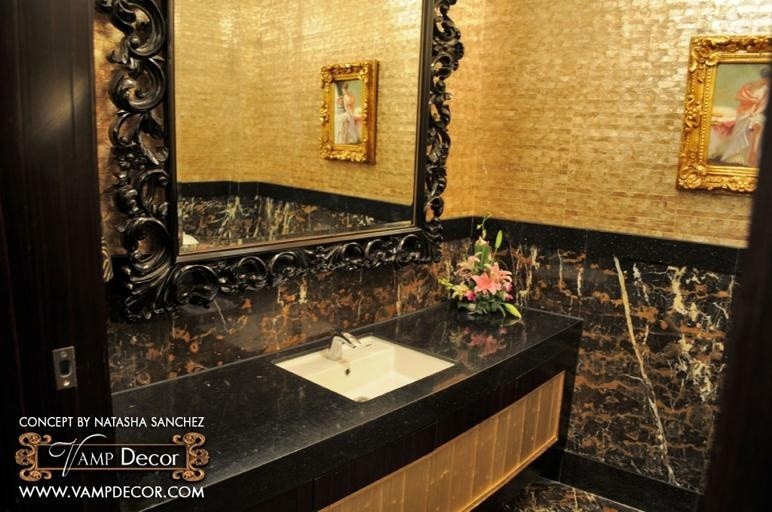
left=674, top=33, right=772, bottom=197
left=316, top=58, right=379, bottom=167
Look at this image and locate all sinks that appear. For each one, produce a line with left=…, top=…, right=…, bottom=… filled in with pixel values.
left=270, top=333, right=460, bottom=404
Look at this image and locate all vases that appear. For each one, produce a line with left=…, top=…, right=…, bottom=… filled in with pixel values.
left=461, top=298, right=498, bottom=314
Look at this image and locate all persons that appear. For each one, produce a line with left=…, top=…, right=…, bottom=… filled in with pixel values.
left=335, top=83, right=356, bottom=143
left=735, top=68, right=769, bottom=166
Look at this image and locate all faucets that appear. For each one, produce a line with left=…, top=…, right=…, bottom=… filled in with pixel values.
left=329, top=331, right=361, bottom=362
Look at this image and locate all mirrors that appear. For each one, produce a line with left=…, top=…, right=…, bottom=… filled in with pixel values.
left=90, top=0, right=465, bottom=321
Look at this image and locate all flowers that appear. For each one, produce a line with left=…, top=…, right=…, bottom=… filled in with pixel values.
left=436, top=210, right=527, bottom=320
left=447, top=323, right=528, bottom=378
